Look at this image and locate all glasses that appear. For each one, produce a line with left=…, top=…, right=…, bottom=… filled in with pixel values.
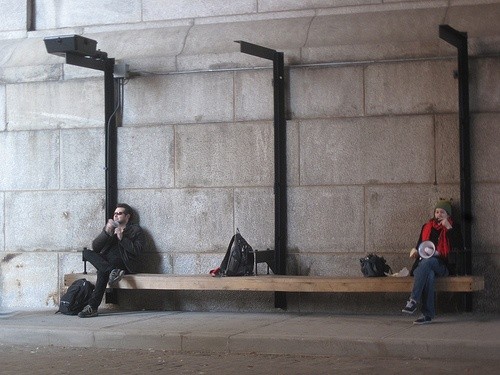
left=114, top=212, right=125, bottom=215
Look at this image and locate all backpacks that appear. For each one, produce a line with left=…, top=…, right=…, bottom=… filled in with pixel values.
left=218, top=234, right=254, bottom=276
left=59, top=279, right=93, bottom=315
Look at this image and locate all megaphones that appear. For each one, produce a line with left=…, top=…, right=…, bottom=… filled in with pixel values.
left=418, top=240, right=441, bottom=259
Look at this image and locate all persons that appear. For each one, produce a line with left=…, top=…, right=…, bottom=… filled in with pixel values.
left=76, top=202, right=141, bottom=318
left=400, top=201, right=462, bottom=325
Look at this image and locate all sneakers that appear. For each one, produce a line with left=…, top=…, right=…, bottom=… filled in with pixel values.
left=413, top=315, right=432, bottom=325
left=402, top=300, right=417, bottom=314
left=108, top=269, right=125, bottom=286
left=77, top=304, right=98, bottom=317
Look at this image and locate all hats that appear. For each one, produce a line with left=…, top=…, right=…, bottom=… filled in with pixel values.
left=436, top=200, right=454, bottom=216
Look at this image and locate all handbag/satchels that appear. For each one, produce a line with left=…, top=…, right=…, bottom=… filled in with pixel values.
left=361, top=256, right=386, bottom=277
